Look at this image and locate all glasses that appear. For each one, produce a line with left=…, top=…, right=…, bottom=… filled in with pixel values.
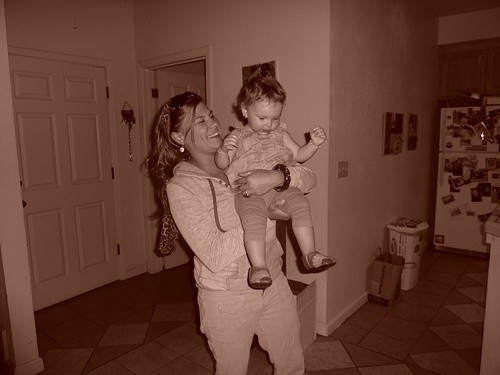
left=170, top=91, right=197, bottom=120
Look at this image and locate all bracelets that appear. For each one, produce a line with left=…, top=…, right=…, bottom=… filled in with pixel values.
left=271, top=163, right=292, bottom=192
left=217, top=147, right=229, bottom=155
left=310, top=140, right=321, bottom=149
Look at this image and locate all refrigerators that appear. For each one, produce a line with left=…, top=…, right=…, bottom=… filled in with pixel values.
left=434, top=106, right=500, bottom=258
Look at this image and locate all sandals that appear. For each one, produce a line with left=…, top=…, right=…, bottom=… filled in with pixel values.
left=248, top=267, right=272, bottom=289
left=302, top=250, right=336, bottom=273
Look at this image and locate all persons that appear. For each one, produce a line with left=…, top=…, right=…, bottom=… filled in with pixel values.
left=214, top=78, right=338, bottom=290
left=145, top=91, right=306, bottom=375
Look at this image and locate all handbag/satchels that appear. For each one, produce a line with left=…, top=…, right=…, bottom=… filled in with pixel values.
left=367, top=247, right=417, bottom=306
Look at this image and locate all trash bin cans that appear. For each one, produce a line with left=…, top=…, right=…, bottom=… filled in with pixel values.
left=386, top=216, right=430, bottom=292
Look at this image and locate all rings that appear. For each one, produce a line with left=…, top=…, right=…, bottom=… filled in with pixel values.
left=245, top=190, right=249, bottom=196
left=247, top=171, right=251, bottom=175
